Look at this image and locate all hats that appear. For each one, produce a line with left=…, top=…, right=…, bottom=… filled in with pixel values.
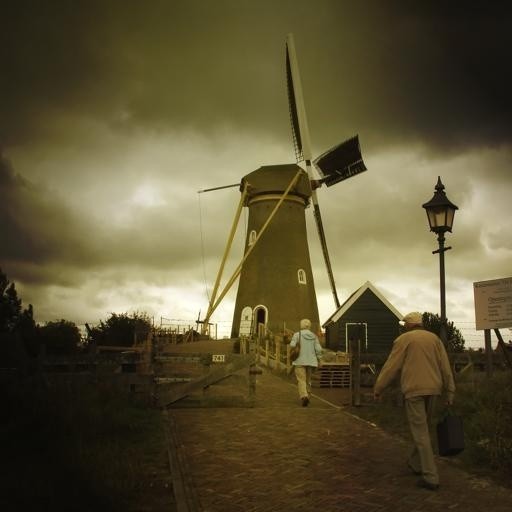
left=301, top=319, right=311, bottom=330
left=402, top=312, right=422, bottom=323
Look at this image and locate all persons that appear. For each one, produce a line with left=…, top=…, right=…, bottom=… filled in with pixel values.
left=288, top=317, right=325, bottom=408
left=371, top=308, right=460, bottom=492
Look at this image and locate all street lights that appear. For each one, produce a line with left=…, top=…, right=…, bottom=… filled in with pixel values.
left=421, top=175, right=459, bottom=350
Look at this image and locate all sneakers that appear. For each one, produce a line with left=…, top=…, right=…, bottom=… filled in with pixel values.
left=303, top=397, right=308, bottom=406
left=417, top=480, right=439, bottom=490
left=407, top=459, right=422, bottom=475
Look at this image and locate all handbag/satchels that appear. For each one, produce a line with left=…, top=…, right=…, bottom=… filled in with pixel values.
left=290, top=342, right=300, bottom=360
left=437, top=416, right=465, bottom=456
